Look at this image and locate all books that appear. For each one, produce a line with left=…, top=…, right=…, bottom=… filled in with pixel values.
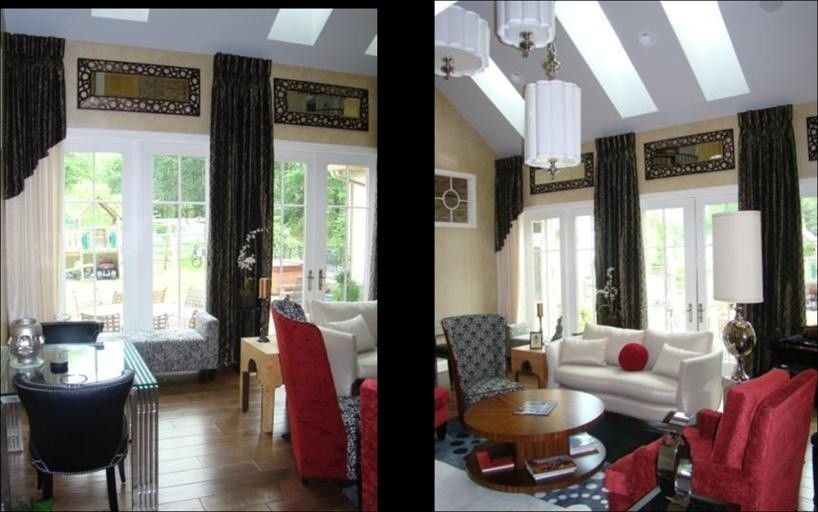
left=525, top=466, right=575, bottom=481
left=476, top=448, right=518, bottom=474
left=568, top=431, right=599, bottom=457
left=524, top=455, right=578, bottom=477
left=513, top=398, right=560, bottom=416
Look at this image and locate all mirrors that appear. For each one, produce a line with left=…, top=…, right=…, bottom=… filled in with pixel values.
left=74, top=56, right=205, bottom=116
left=272, top=74, right=372, bottom=132
left=528, top=151, right=595, bottom=196
left=642, top=128, right=737, bottom=180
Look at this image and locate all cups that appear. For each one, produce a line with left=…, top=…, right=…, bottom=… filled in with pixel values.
left=50, top=347, right=67, bottom=364
left=49, top=361, right=68, bottom=375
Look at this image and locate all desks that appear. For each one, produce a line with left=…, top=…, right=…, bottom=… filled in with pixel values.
left=624, top=485, right=743, bottom=512
left=510, top=343, right=548, bottom=389
left=238, top=335, right=284, bottom=434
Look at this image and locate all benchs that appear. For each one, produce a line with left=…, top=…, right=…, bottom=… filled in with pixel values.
left=121, top=310, right=222, bottom=382
left=507, top=322, right=550, bottom=371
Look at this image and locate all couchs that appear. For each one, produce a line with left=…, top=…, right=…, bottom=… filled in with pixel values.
left=546, top=331, right=724, bottom=426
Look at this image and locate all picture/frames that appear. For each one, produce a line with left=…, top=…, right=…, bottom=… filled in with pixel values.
left=529, top=330, right=544, bottom=350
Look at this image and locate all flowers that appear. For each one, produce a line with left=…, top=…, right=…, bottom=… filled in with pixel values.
left=238, top=225, right=269, bottom=289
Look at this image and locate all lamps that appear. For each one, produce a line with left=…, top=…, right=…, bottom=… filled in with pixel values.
left=492, top=1, right=556, bottom=56
left=709, top=209, right=768, bottom=383
left=524, top=39, right=583, bottom=174
left=434, top=6, right=491, bottom=81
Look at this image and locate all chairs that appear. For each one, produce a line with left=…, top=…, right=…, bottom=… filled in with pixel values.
left=602, top=365, right=817, bottom=511
left=11, top=363, right=138, bottom=512
left=38, top=319, right=104, bottom=345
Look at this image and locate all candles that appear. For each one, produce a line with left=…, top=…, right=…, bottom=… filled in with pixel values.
left=536, top=301, right=544, bottom=317
left=258, top=277, right=271, bottom=300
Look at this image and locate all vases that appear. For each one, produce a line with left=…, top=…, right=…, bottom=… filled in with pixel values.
left=240, top=283, right=255, bottom=309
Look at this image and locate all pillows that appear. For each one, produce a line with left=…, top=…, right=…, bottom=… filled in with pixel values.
left=556, top=319, right=716, bottom=380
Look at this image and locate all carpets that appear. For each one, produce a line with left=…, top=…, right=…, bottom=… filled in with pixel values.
left=340, top=417, right=612, bottom=511
